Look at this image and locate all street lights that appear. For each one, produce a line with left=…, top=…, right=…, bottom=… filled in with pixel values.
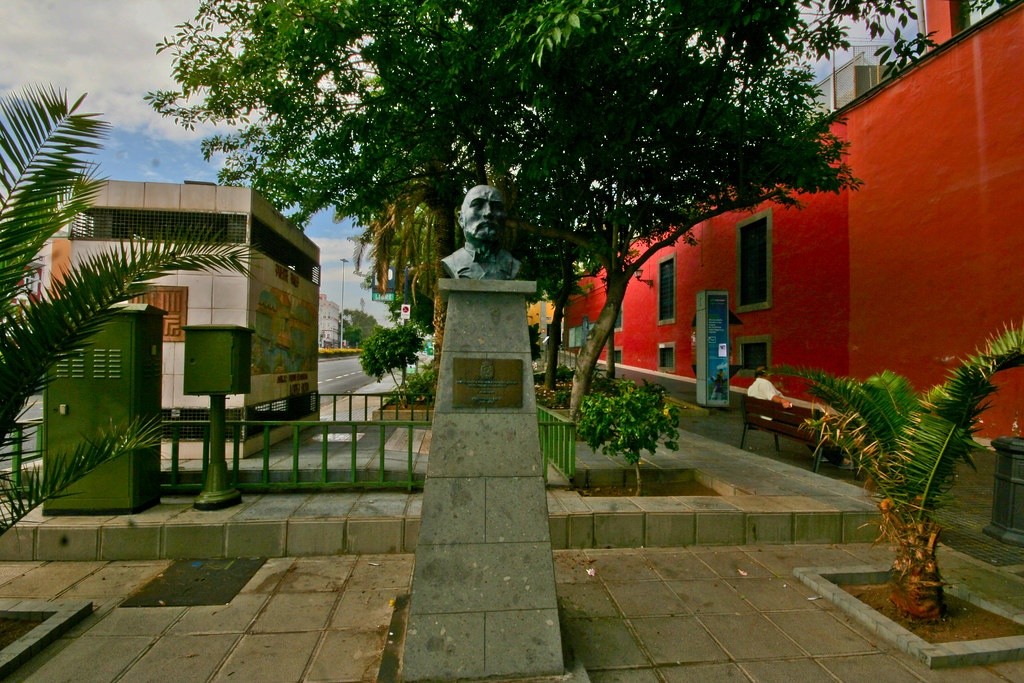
left=340, top=259, right=349, bottom=349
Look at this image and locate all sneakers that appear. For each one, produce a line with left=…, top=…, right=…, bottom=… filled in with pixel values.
left=837, top=460, right=863, bottom=469
left=820, top=456, right=830, bottom=462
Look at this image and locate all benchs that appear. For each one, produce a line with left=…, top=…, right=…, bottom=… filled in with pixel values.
left=738, top=392, right=861, bottom=481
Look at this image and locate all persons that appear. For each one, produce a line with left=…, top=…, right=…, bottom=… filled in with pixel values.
left=441, top=184, right=523, bottom=278
left=710, top=369, right=728, bottom=401
left=748, top=365, right=864, bottom=472
